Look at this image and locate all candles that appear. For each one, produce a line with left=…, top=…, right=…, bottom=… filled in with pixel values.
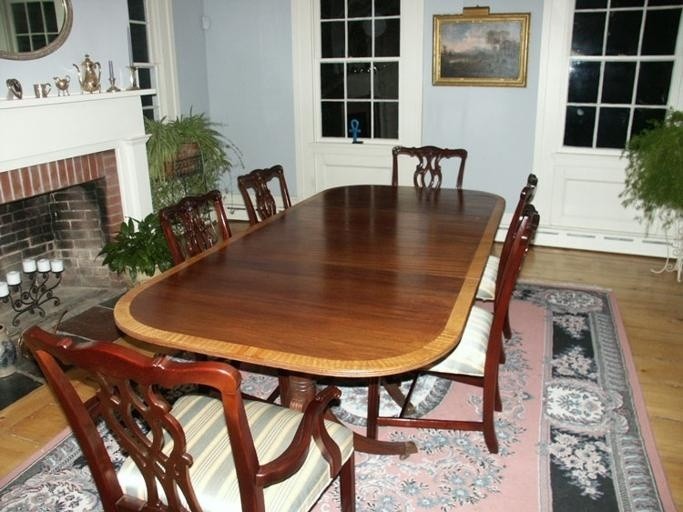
left=0, top=255, right=65, bottom=297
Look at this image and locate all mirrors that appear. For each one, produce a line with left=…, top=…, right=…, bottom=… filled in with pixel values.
left=0, top=0, right=72, bottom=61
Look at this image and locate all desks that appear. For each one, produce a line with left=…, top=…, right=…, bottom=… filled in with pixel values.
left=110, top=183, right=506, bottom=470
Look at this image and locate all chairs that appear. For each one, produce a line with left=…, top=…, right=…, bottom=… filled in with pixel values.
left=20, top=324, right=360, bottom=512
left=365, top=218, right=534, bottom=457
left=472, top=174, right=539, bottom=361
left=390, top=143, right=466, bottom=192
left=156, top=165, right=294, bottom=264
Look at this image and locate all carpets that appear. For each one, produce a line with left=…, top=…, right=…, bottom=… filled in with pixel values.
left=1, top=279, right=674, bottom=512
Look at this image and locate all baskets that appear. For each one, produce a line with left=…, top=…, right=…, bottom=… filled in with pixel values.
left=164, top=143, right=201, bottom=178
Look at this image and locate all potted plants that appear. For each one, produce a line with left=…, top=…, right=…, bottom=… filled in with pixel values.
left=94, top=211, right=186, bottom=290
left=142, top=105, right=251, bottom=223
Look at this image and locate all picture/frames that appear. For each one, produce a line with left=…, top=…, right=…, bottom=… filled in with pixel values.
left=429, top=13, right=529, bottom=89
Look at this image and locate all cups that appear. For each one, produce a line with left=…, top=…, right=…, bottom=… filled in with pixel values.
left=33, top=83, right=52, bottom=99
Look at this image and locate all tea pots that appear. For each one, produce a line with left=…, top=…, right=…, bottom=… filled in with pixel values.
left=73, top=54, right=102, bottom=95
